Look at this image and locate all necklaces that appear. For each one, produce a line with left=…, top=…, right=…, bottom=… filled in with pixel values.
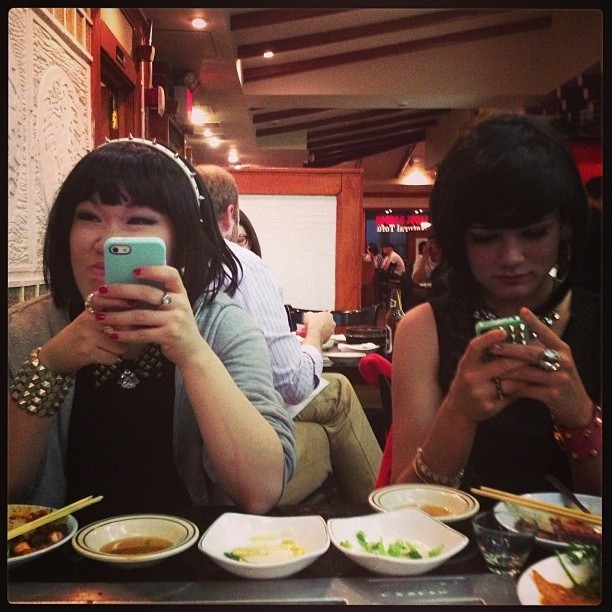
left=95, top=341, right=161, bottom=389
left=466, top=276, right=570, bottom=341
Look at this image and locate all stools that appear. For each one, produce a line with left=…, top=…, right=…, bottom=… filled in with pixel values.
left=385, top=279, right=407, bottom=321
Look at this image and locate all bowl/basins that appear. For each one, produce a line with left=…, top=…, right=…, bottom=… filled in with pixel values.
left=345, top=326, right=386, bottom=349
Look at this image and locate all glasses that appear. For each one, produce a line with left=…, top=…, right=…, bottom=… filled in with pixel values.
left=237, top=235, right=249, bottom=247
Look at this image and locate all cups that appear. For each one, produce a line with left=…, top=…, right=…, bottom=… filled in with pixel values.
left=472, top=511, right=540, bottom=577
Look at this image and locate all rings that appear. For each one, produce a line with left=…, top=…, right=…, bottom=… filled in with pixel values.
left=85, top=290, right=102, bottom=315
left=539, top=348, right=561, bottom=372
left=103, top=323, right=116, bottom=336
left=158, top=290, right=173, bottom=308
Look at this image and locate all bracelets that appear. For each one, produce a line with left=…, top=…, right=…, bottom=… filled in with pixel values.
left=413, top=460, right=428, bottom=494
left=551, top=402, right=602, bottom=461
left=415, top=446, right=465, bottom=485
left=9, top=345, right=76, bottom=419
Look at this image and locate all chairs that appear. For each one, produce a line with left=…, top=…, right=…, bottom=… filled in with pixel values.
left=285, top=302, right=392, bottom=438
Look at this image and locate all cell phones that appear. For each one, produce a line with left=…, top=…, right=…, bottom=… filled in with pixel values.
left=475, top=316, right=526, bottom=344
left=104, top=237, right=167, bottom=286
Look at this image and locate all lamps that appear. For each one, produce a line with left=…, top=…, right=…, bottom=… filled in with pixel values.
left=396, top=157, right=435, bottom=186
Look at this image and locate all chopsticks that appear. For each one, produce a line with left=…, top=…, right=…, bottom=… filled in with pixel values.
left=7, top=494, right=103, bottom=540
left=470, top=485, right=602, bottom=526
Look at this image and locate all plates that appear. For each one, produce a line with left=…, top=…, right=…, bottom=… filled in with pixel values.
left=338, top=341, right=381, bottom=354
left=368, top=483, right=480, bottom=524
left=326, top=506, right=470, bottom=578
left=6, top=504, right=78, bottom=570
left=198, top=512, right=330, bottom=581
left=516, top=551, right=603, bottom=606
left=494, top=491, right=602, bottom=551
left=72, top=513, right=200, bottom=570
left=325, top=352, right=366, bottom=367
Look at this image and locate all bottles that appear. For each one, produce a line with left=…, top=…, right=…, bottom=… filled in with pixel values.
left=385, top=280, right=406, bottom=359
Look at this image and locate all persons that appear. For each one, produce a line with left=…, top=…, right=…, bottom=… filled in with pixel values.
left=378, top=243, right=407, bottom=299
left=184, top=163, right=385, bottom=515
left=578, top=175, right=603, bottom=295
left=387, top=108, right=603, bottom=500
left=8, top=136, right=296, bottom=519
left=233, top=207, right=263, bottom=261
left=411, top=235, right=443, bottom=285
left=362, top=243, right=384, bottom=284
